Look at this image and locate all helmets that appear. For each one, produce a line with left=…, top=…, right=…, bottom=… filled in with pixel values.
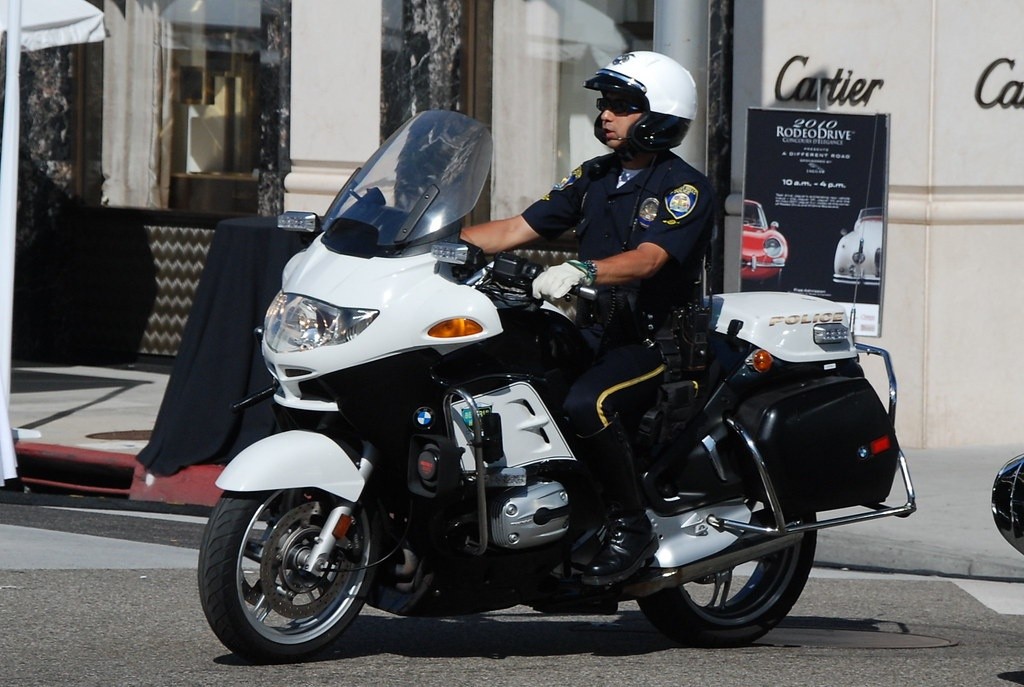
left=581, top=50, right=699, bottom=152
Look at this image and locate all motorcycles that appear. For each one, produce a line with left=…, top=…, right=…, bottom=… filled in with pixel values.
left=194, top=109, right=916, bottom=664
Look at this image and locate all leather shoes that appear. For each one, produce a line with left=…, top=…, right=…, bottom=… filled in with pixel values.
left=582, top=514, right=660, bottom=587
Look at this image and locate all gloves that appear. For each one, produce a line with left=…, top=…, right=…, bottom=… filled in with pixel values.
left=531, top=258, right=594, bottom=300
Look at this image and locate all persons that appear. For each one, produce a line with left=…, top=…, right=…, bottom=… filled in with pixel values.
left=461, top=48, right=715, bottom=586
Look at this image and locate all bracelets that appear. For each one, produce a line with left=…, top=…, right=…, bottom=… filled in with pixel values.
left=569, top=258, right=597, bottom=286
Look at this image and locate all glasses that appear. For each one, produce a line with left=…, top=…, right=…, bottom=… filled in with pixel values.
left=595, top=97, right=646, bottom=116
